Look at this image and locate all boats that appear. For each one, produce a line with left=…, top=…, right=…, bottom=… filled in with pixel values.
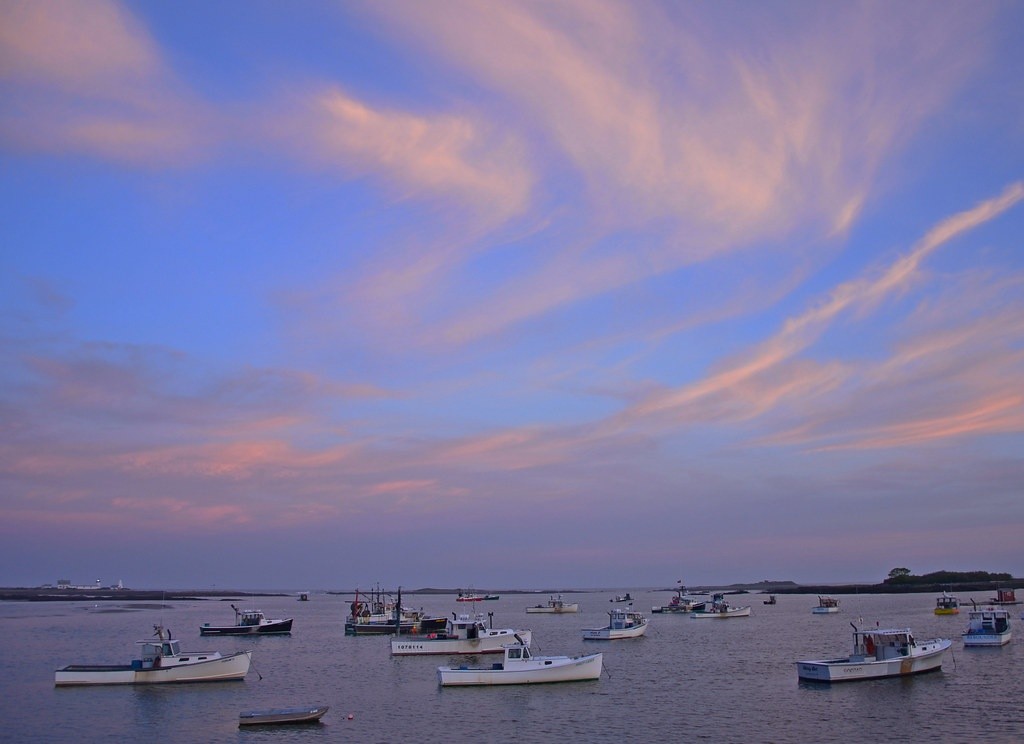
left=340, top=585, right=447, bottom=635
left=809, top=595, right=840, bottom=614
left=796, top=616, right=954, bottom=684
left=652, top=580, right=701, bottom=614
left=455, top=586, right=483, bottom=602
left=300, top=593, right=308, bottom=601
left=581, top=606, right=650, bottom=640
left=481, top=591, right=500, bottom=601
left=54, top=588, right=253, bottom=684
left=609, top=592, right=631, bottom=604
left=990, top=584, right=1024, bottom=604
left=763, top=595, right=776, bottom=606
left=688, top=592, right=751, bottom=618
left=435, top=631, right=603, bottom=686
left=934, top=591, right=962, bottom=615
left=238, top=703, right=331, bottom=725
left=387, top=596, right=533, bottom=658
left=200, top=603, right=294, bottom=635
left=524, top=593, right=578, bottom=613
left=959, top=597, right=1012, bottom=646
